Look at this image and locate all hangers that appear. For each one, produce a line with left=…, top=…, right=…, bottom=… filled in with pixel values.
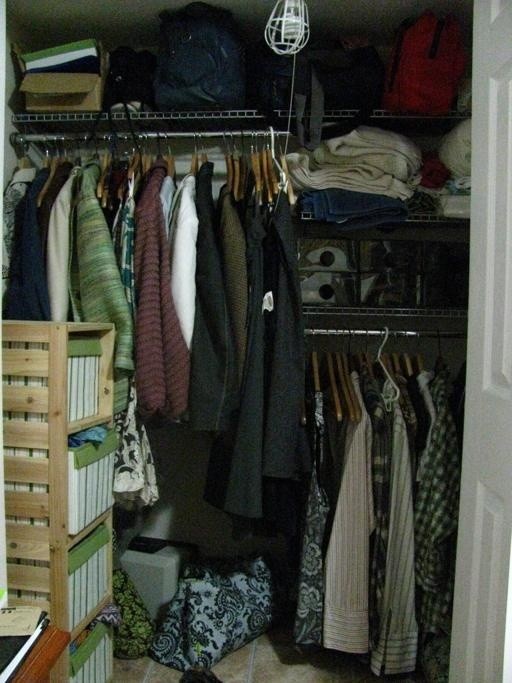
left=15, top=130, right=297, bottom=207
left=307, top=327, right=447, bottom=425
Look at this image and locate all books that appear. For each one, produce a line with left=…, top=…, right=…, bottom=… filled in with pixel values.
left=0, top=604, right=52, bottom=682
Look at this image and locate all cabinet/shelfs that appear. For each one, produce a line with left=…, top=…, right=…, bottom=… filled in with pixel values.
left=0, top=319, right=115, bottom=683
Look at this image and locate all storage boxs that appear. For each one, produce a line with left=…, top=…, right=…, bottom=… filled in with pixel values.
left=121, top=545, right=180, bottom=623
left=12, top=41, right=110, bottom=113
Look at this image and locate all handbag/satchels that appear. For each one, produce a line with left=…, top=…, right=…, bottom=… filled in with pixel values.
left=250, top=31, right=312, bottom=112
left=105, top=45, right=156, bottom=114
left=384, top=3, right=466, bottom=116
left=153, top=2, right=248, bottom=112
left=312, top=45, right=384, bottom=116
